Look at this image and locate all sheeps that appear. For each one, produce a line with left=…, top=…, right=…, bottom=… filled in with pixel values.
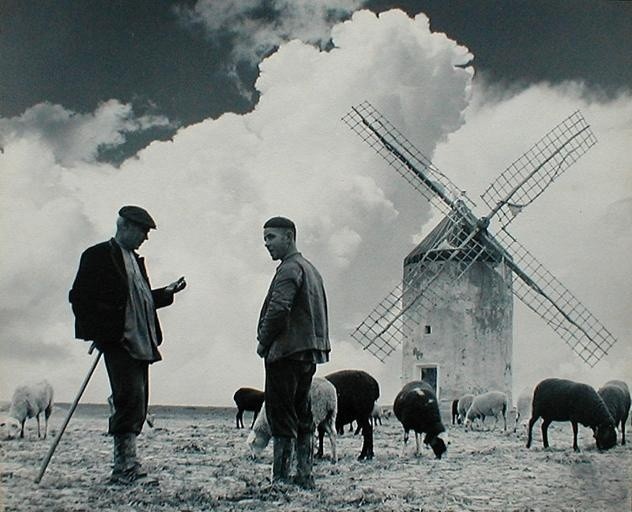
left=391, top=379, right=451, bottom=460
left=0, top=372, right=55, bottom=440
left=451, top=392, right=511, bottom=432
left=526, top=376, right=614, bottom=452
left=513, top=386, right=535, bottom=430
left=597, top=378, right=632, bottom=447
left=230, top=366, right=386, bottom=466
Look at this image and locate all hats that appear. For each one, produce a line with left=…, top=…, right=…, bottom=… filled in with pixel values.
left=118, top=205, right=158, bottom=231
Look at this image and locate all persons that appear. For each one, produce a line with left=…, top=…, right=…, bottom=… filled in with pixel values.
left=254, top=214, right=332, bottom=492
left=68, top=205, right=188, bottom=489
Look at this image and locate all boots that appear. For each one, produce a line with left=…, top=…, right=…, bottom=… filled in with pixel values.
left=288, top=431, right=316, bottom=491
left=271, top=436, right=296, bottom=487
left=110, top=432, right=161, bottom=487
